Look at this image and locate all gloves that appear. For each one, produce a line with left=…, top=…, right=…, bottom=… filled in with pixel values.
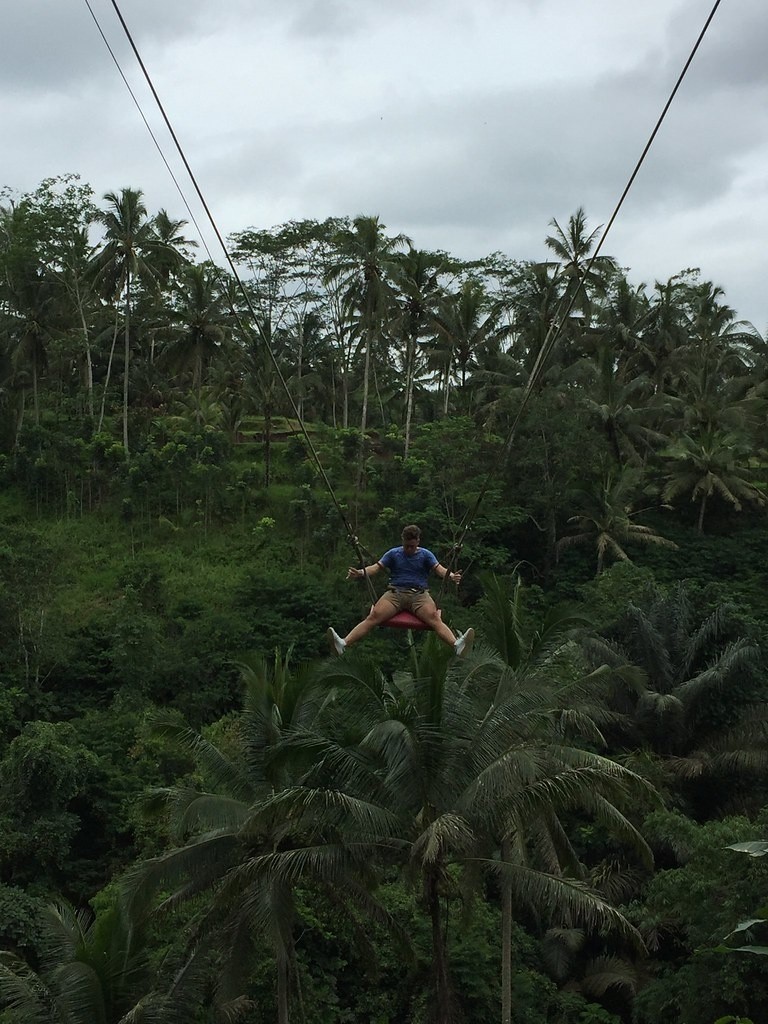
left=345, top=567, right=365, bottom=580
left=449, top=571, right=461, bottom=585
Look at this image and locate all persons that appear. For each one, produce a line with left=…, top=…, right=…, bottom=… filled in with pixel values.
left=327, top=525, right=474, bottom=657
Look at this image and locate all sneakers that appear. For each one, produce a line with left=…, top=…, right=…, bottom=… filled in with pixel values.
left=454, top=627, right=475, bottom=659
left=326, top=627, right=347, bottom=658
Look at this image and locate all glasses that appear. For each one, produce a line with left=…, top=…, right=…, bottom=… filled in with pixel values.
left=404, top=544, right=415, bottom=549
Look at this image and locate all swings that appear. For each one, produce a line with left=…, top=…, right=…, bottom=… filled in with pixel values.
left=88, top=0, right=724, bottom=634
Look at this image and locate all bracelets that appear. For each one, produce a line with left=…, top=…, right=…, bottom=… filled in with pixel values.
left=362, top=570, right=364, bottom=576
left=450, top=574, right=452, bottom=580
